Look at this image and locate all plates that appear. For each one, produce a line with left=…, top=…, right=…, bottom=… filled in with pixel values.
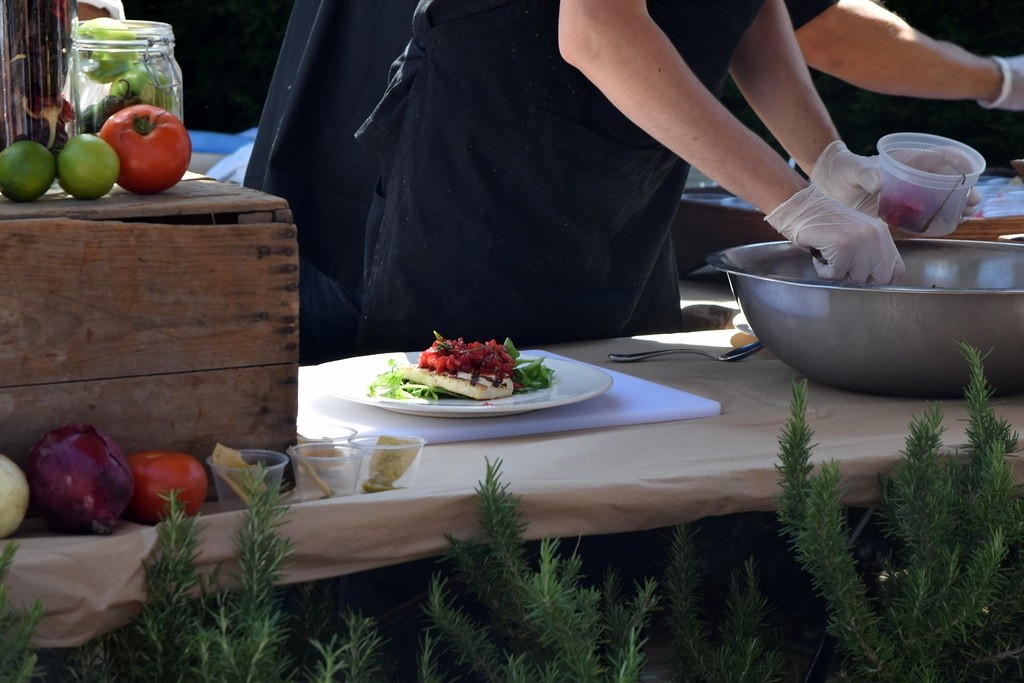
left=341, top=357, right=614, bottom=418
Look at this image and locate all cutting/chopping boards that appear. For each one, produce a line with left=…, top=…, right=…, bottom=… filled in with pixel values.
left=298, top=350, right=721, bottom=448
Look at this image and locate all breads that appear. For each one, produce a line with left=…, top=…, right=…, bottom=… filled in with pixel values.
left=398, top=364, right=514, bottom=400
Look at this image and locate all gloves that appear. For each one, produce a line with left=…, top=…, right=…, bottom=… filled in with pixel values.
left=811, top=140, right=981, bottom=225
left=763, top=181, right=905, bottom=287
left=978, top=54, right=1024, bottom=110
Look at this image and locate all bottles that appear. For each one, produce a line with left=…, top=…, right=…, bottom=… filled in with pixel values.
left=70, top=18, right=184, bottom=173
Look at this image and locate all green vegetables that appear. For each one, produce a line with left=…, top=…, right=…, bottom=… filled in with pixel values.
left=369, top=337, right=555, bottom=401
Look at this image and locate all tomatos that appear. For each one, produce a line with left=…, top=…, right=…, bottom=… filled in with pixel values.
left=123, top=449, right=208, bottom=525
left=100, top=103, right=191, bottom=195
left=76, top=18, right=174, bottom=111
left=0, top=140, right=55, bottom=202
left=58, top=133, right=120, bottom=201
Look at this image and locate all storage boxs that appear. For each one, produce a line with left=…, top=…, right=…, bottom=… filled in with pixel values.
left=0, top=169, right=299, bottom=501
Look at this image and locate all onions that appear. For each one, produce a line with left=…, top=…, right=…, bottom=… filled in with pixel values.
left=26, top=422, right=135, bottom=536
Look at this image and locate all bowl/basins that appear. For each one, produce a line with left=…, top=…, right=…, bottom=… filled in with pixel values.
left=347, top=433, right=427, bottom=492
left=206, top=450, right=290, bottom=509
left=877, top=131, right=986, bottom=237
left=706, top=237, right=1023, bottom=397
left=286, top=442, right=364, bottom=501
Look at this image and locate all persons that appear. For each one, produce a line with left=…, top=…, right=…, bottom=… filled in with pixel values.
left=243, top=2, right=1023, bottom=366
left=355, top=0, right=907, bottom=359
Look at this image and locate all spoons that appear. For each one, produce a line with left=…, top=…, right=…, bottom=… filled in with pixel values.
left=609, top=339, right=765, bottom=361
